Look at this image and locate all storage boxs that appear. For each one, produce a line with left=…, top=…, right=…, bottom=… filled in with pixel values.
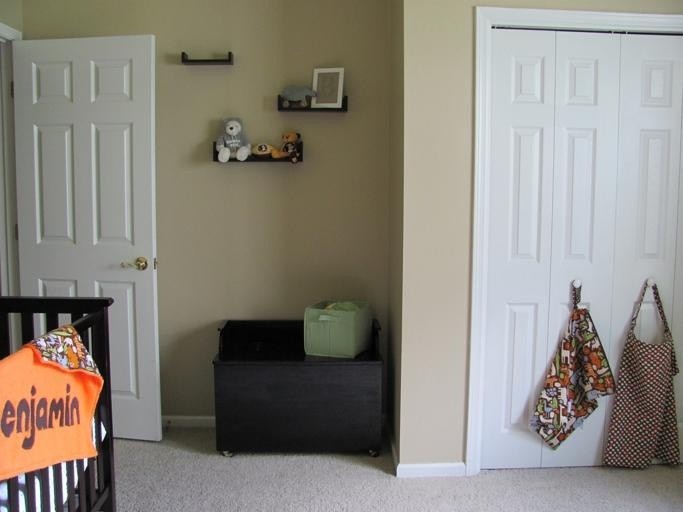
left=303, top=298, right=373, bottom=359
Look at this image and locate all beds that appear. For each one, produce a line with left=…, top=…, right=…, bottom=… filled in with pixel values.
left=0, top=295, right=117, bottom=512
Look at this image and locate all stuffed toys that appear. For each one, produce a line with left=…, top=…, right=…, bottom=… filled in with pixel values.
left=217, top=118, right=250, bottom=163
left=272, top=128, right=301, bottom=164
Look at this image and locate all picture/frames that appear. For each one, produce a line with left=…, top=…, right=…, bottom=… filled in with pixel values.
left=310, top=67, right=344, bottom=108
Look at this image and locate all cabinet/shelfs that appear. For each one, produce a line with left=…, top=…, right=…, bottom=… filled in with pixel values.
left=181, top=51, right=348, bottom=162
